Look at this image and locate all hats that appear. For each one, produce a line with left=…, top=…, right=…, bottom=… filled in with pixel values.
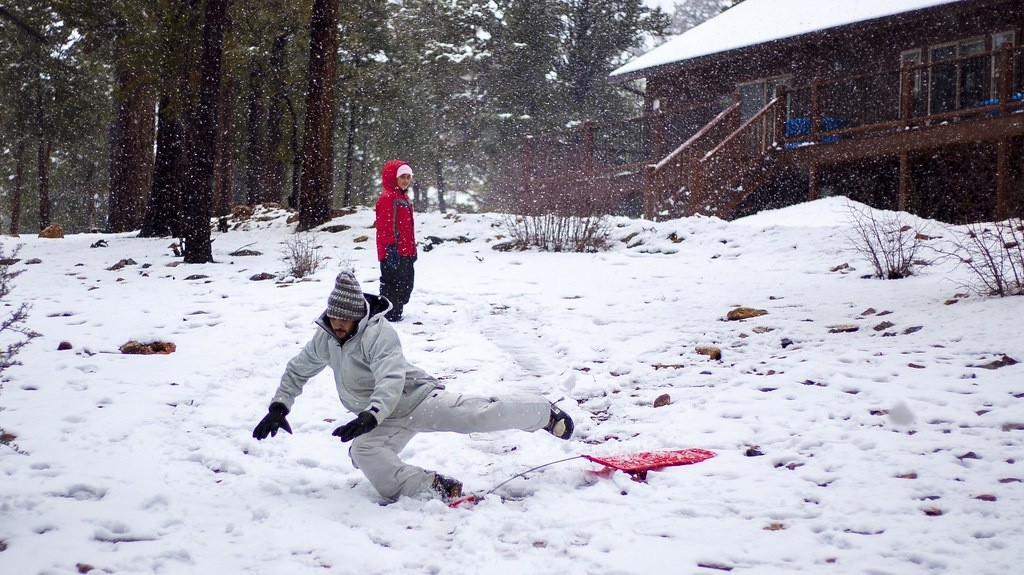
left=396, top=164, right=412, bottom=179
left=327, top=271, right=366, bottom=321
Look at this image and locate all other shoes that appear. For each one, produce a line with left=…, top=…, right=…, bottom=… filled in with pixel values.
left=384, top=315, right=404, bottom=322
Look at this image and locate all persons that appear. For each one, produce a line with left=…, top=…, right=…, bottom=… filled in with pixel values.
left=253, top=271, right=574, bottom=503
left=374, top=159, right=417, bottom=322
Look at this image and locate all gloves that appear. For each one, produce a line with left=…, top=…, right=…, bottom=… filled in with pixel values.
left=332, top=411, right=378, bottom=442
left=253, top=401, right=293, bottom=440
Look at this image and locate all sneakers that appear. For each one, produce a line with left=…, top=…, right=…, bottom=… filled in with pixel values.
left=542, top=400, right=574, bottom=440
left=431, top=474, right=463, bottom=503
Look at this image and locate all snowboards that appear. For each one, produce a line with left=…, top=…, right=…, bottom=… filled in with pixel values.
left=580, top=448, right=717, bottom=472
